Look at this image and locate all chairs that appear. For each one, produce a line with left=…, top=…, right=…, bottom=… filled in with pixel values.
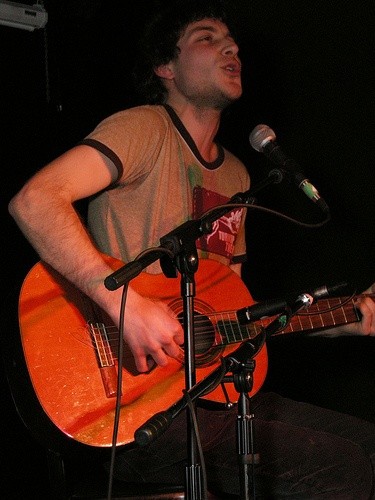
left=2, top=327, right=116, bottom=499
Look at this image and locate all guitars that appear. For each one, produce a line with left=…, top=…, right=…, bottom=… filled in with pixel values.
left=4, top=252, right=375, bottom=458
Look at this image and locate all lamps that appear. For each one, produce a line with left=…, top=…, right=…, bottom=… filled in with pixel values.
left=1, top=3, right=48, bottom=31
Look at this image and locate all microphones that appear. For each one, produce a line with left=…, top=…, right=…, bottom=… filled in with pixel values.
left=249, top=125, right=328, bottom=211
left=237, top=282, right=347, bottom=325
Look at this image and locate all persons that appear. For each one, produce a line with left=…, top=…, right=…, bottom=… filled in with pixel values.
left=8, top=0, right=375, bottom=500
